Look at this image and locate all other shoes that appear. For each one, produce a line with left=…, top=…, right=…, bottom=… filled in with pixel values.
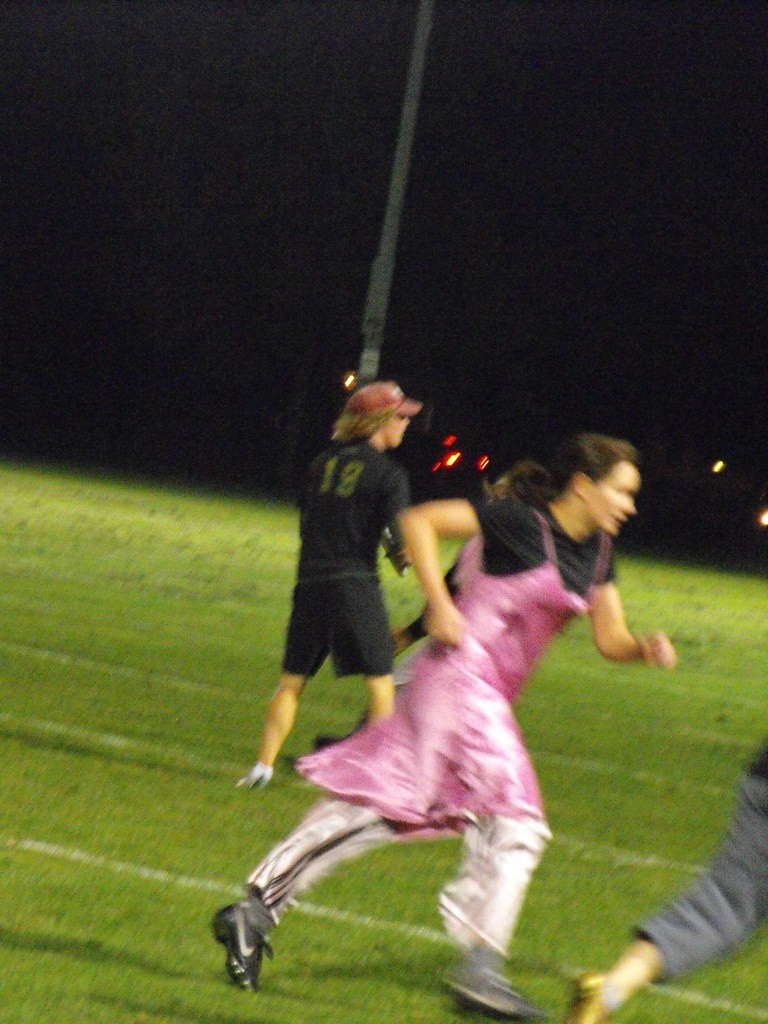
left=235, top=764, right=276, bottom=796
left=563, top=966, right=611, bottom=1023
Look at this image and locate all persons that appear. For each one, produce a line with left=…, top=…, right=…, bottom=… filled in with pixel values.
left=212, top=437, right=677, bottom=1024
left=235, top=381, right=423, bottom=789
left=562, top=731, right=768, bottom=1024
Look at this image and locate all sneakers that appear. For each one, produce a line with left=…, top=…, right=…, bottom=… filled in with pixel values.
left=204, top=899, right=273, bottom=994
left=446, top=960, right=544, bottom=1022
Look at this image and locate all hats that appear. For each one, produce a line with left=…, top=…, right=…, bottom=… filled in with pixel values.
left=351, top=379, right=427, bottom=422
